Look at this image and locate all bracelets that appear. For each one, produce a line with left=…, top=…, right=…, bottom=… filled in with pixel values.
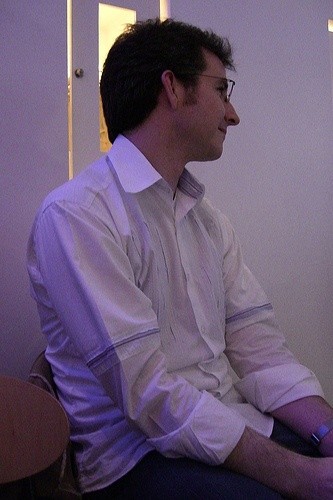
left=24, top=18, right=333, bottom=500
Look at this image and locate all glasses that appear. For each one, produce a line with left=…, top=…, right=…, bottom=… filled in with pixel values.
left=173, top=72, right=235, bottom=102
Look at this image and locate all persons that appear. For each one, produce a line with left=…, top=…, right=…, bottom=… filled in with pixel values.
left=307, top=417, right=333, bottom=448
